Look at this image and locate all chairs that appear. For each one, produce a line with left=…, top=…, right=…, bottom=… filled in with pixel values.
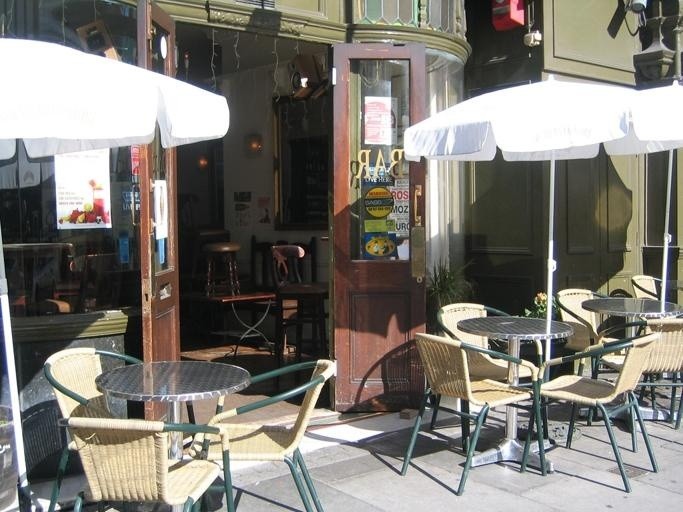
left=249, top=233, right=317, bottom=360
left=1, top=242, right=138, bottom=316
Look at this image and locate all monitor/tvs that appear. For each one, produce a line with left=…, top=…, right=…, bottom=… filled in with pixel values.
left=117, top=235, right=166, bottom=270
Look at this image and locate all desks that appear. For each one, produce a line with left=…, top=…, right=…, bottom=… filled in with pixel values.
left=180, top=282, right=329, bottom=362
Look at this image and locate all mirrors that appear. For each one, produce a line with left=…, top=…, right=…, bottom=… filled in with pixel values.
left=270, top=90, right=330, bottom=232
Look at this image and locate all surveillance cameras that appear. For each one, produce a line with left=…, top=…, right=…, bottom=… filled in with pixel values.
left=627, top=0, right=649, bottom=13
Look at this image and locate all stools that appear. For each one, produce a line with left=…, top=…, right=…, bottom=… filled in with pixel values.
left=201, top=242, right=241, bottom=297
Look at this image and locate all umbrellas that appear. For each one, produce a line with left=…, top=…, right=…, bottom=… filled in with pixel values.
left=0, top=34, right=235, bottom=512
left=638, top=77, right=682, bottom=316
left=398, top=70, right=682, bottom=429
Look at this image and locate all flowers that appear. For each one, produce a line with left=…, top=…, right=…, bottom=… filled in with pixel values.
left=524, top=291, right=559, bottom=320
